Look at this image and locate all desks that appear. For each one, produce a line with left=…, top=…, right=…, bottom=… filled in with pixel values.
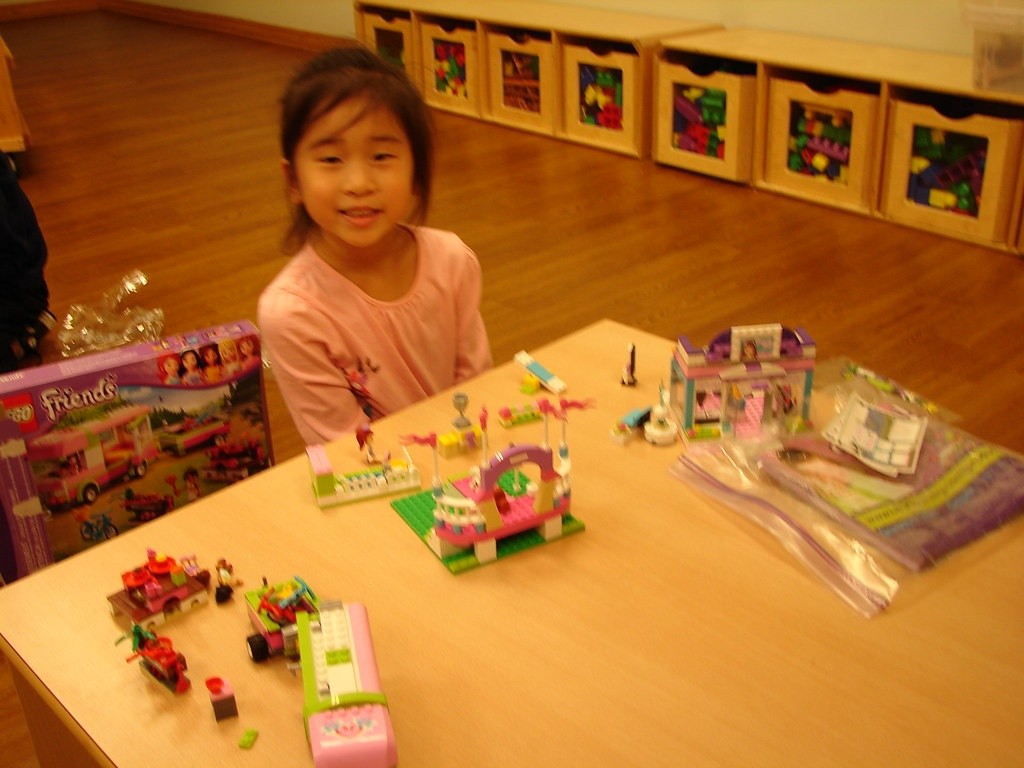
left=0, top=317, right=1021, bottom=767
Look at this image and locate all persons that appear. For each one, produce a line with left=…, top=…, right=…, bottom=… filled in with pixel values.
left=256, top=46, right=491, bottom=450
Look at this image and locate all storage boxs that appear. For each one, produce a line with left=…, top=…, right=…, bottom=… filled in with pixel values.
left=652, top=26, right=1023, bottom=257
left=353, top=0, right=725, bottom=161
